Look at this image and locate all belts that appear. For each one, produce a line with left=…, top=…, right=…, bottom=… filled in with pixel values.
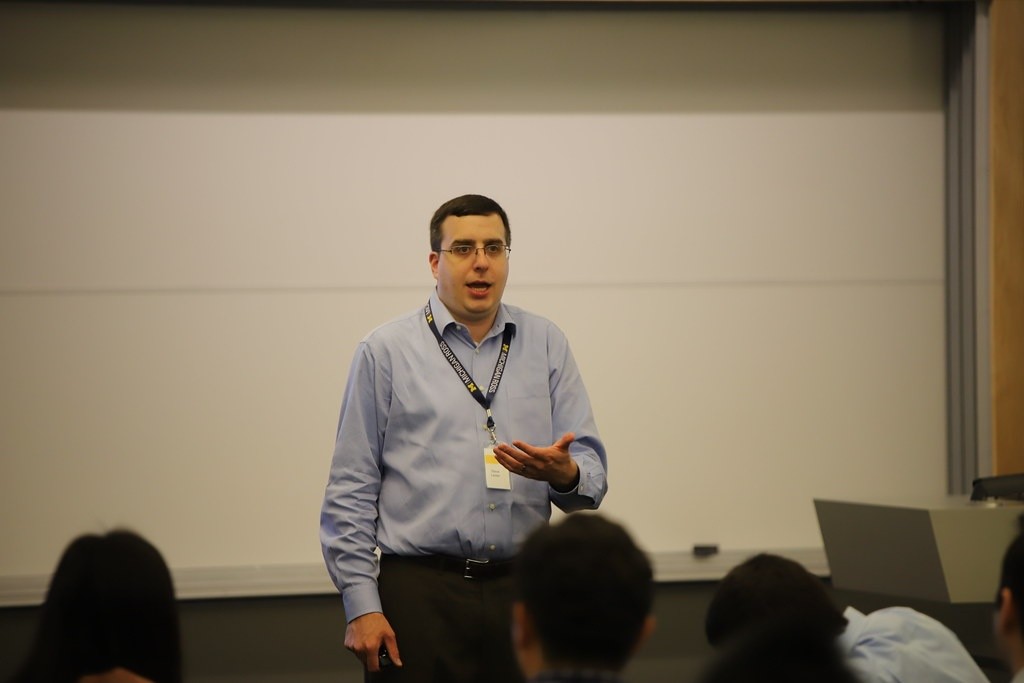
left=411, top=552, right=519, bottom=578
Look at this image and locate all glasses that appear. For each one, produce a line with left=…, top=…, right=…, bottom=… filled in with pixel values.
left=435, top=245, right=511, bottom=262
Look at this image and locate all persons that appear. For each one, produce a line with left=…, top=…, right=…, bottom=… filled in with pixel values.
left=318, top=195, right=608, bottom=681
left=688, top=528, right=1024, bottom=683
left=509, top=513, right=658, bottom=677
left=6, top=528, right=191, bottom=683
